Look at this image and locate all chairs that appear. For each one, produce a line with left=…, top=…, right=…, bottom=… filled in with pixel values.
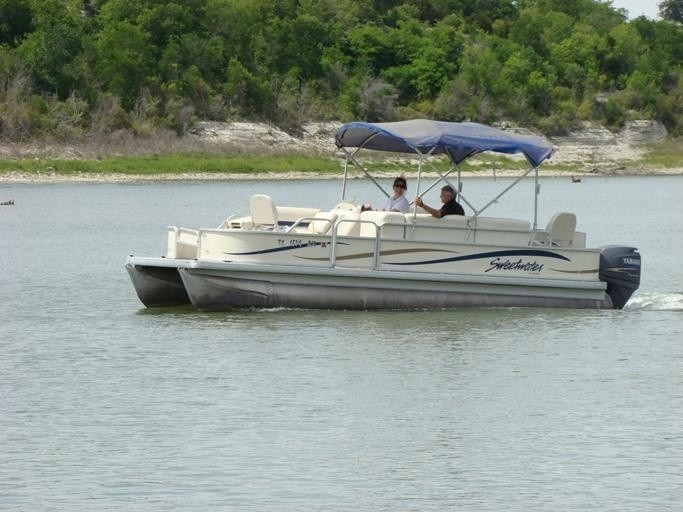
left=536, top=212, right=576, bottom=248
left=308, top=211, right=338, bottom=232
left=249, top=193, right=281, bottom=232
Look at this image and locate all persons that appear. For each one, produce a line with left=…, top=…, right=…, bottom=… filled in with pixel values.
left=414, top=186, right=465, bottom=219
left=362, top=175, right=409, bottom=213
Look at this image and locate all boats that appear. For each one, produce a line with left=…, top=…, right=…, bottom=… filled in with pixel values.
left=123, top=119, right=642, bottom=310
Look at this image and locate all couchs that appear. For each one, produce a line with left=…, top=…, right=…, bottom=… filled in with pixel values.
left=360, top=209, right=533, bottom=245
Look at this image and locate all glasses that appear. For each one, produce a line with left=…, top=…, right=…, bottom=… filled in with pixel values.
left=395, top=184, right=405, bottom=188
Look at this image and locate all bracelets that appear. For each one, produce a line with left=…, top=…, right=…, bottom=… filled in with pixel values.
left=420, top=201, right=423, bottom=207
